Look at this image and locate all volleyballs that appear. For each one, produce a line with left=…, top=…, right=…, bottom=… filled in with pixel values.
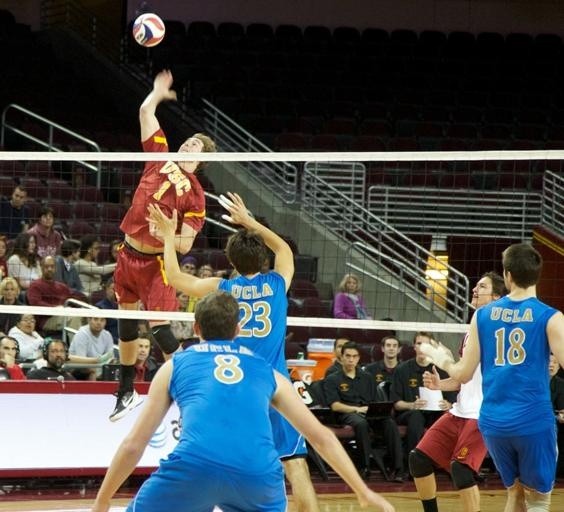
left=133, top=13, right=165, bottom=47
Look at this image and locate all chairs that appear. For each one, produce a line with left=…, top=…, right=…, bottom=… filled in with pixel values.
left=0, top=116, right=564, bottom=481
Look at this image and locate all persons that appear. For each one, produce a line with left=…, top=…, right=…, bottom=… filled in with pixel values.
left=1, top=184, right=275, bottom=383
left=109, top=69, right=217, bottom=422
left=323, top=243, right=564, bottom=512
left=92, top=290, right=395, bottom=512
left=145, top=191, right=321, bottom=512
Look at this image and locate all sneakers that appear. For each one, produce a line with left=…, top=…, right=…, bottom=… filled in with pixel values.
left=359, top=467, right=405, bottom=483
left=109, top=387, right=144, bottom=422
